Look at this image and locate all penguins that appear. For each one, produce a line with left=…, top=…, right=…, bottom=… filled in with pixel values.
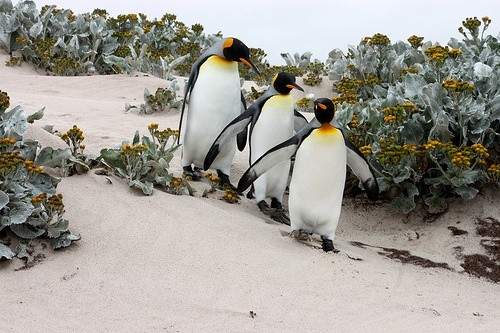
left=236, top=97, right=379, bottom=253
left=204, top=72, right=308, bottom=225
left=177, top=37, right=261, bottom=197
